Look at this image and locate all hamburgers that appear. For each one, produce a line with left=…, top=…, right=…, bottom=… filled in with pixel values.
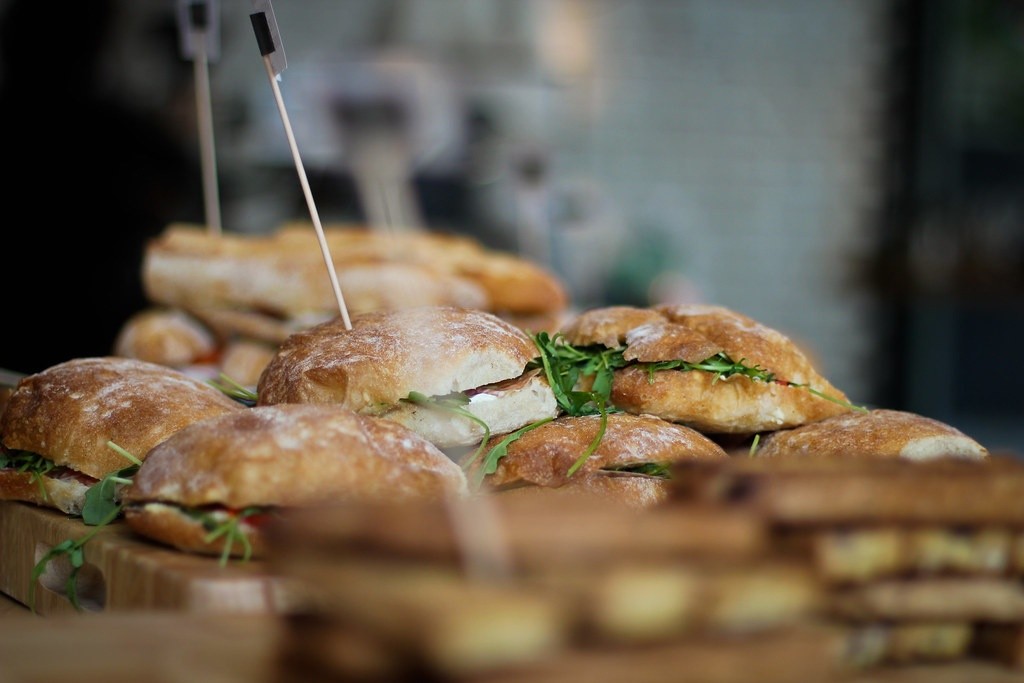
left=0, top=304, right=1024, bottom=683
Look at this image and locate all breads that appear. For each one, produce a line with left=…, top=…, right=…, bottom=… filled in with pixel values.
left=115, top=218, right=566, bottom=400
